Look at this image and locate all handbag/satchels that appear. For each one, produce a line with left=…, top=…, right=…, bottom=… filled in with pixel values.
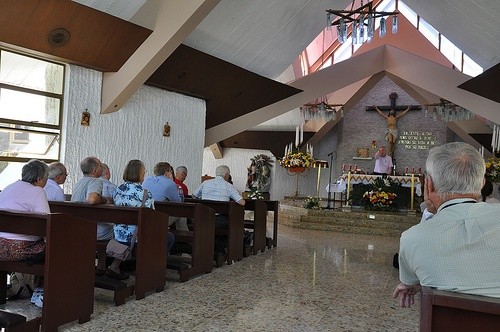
left=106, top=238, right=131, bottom=262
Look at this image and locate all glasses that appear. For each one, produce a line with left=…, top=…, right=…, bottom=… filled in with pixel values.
left=56, top=173, right=68, bottom=178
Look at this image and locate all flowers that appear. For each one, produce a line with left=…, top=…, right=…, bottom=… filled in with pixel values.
left=363, top=191, right=399, bottom=207
left=485, top=158, right=500, bottom=183
left=279, top=154, right=317, bottom=170
left=301, top=195, right=322, bottom=210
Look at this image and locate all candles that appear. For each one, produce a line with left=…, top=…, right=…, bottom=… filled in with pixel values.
left=355, top=164, right=358, bottom=170
left=411, top=166, right=415, bottom=173
left=475, top=124, right=500, bottom=158
left=348, top=164, right=352, bottom=171
left=285, top=124, right=314, bottom=157
left=342, top=163, right=346, bottom=172
left=405, top=167, right=408, bottom=173
left=418, top=168, right=421, bottom=173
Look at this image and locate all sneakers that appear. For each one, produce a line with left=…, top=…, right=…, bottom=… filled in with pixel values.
left=30, top=292, right=41, bottom=303
left=35, top=296, right=44, bottom=308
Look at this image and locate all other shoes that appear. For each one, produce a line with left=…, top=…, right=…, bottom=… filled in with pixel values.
left=105, top=268, right=130, bottom=280
left=95, top=268, right=106, bottom=275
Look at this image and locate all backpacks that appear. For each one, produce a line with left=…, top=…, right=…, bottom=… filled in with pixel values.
left=7, top=272, right=33, bottom=300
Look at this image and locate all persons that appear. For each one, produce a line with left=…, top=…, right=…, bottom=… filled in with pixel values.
left=0, top=158, right=51, bottom=307
left=176, top=166, right=187, bottom=182
left=373, top=146, right=393, bottom=175
left=192, top=165, right=245, bottom=227
left=98, top=163, right=118, bottom=197
left=43, top=162, right=68, bottom=201
left=142, top=162, right=189, bottom=231
left=169, top=166, right=188, bottom=196
left=393, top=142, right=500, bottom=308
left=375, top=106, right=411, bottom=157
left=417, top=180, right=500, bottom=223
left=71, top=157, right=129, bottom=280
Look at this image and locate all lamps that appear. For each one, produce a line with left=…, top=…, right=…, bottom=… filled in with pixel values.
left=424, top=98, right=475, bottom=123
left=300, top=95, right=345, bottom=124
left=324, top=0, right=400, bottom=45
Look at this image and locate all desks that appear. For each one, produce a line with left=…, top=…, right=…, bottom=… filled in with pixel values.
left=340, top=173, right=422, bottom=215
left=326, top=183, right=343, bottom=209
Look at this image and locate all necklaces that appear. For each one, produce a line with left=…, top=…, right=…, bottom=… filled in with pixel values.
left=440, top=200, right=477, bottom=210
left=112, top=160, right=175, bottom=259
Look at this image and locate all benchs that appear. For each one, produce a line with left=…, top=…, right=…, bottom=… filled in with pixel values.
left=243, top=199, right=268, bottom=255
left=157, top=202, right=217, bottom=282
left=53, top=201, right=169, bottom=306
left=0, top=208, right=97, bottom=332
left=193, top=200, right=244, bottom=265
left=265, top=200, right=279, bottom=250
left=419, top=287, right=500, bottom=332
